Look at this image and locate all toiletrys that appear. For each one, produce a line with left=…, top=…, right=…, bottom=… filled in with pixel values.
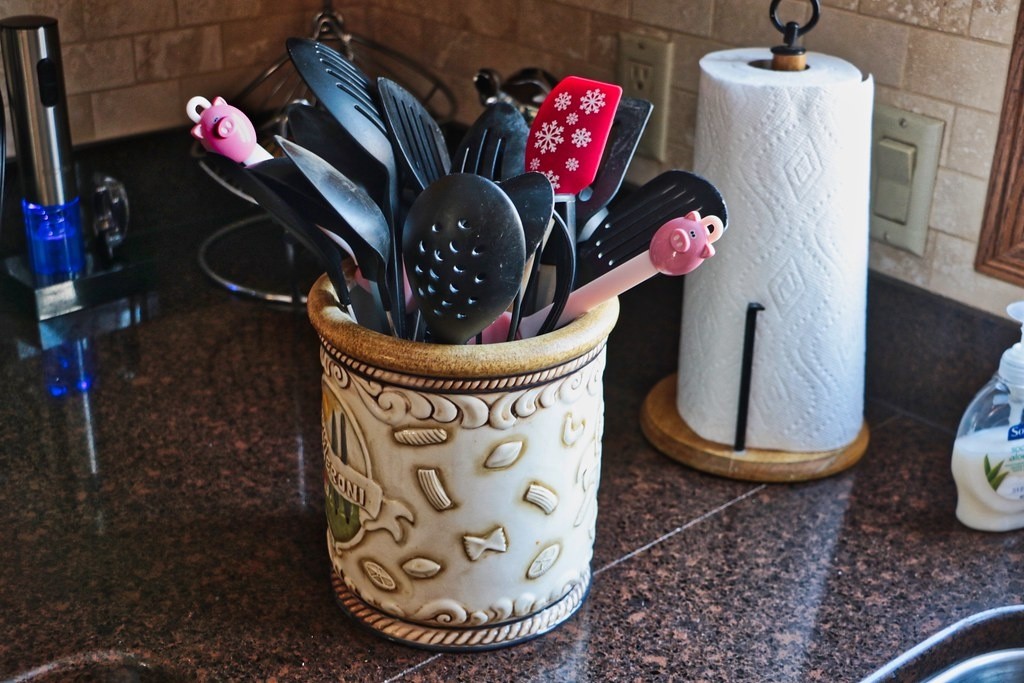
left=948, top=301, right=1022, bottom=532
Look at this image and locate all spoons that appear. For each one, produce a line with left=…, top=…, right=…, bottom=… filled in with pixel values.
left=249, top=135, right=393, bottom=334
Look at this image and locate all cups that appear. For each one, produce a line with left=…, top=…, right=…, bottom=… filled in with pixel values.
left=308, top=266, right=619, bottom=650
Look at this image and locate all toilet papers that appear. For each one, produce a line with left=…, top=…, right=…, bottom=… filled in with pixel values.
left=673, top=43, right=881, bottom=455
